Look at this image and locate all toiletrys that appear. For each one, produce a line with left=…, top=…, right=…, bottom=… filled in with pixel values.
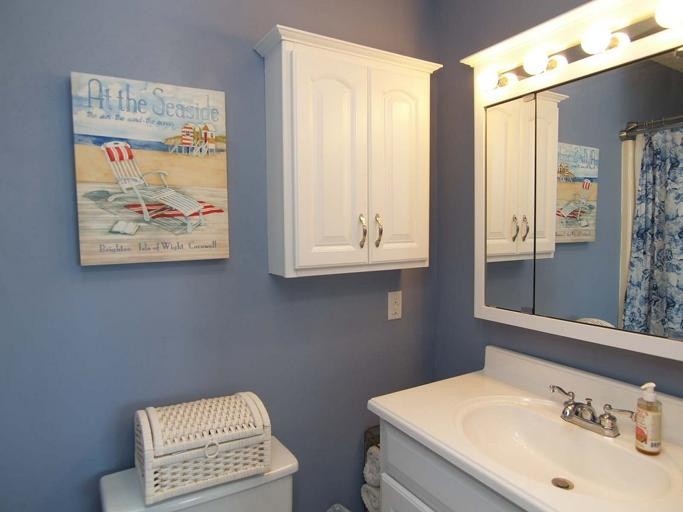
left=635, top=382, right=662, bottom=456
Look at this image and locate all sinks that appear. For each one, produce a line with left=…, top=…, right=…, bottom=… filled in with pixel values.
left=453, top=395, right=683, bottom=512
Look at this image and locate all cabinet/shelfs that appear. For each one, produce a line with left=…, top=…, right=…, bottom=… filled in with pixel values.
left=484, top=88, right=571, bottom=263
left=254, top=24, right=442, bottom=281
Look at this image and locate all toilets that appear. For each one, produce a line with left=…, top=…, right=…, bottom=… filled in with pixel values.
left=99, top=434, right=299, bottom=512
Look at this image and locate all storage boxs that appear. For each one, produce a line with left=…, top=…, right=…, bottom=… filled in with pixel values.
left=130, top=385, right=274, bottom=508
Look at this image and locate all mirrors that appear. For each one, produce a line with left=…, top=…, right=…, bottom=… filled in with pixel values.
left=458, top=35, right=682, bottom=362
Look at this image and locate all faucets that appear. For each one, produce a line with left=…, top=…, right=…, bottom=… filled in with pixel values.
left=561, top=398, right=597, bottom=422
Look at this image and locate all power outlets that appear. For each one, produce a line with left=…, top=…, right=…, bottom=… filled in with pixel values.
left=387, top=291, right=403, bottom=321
